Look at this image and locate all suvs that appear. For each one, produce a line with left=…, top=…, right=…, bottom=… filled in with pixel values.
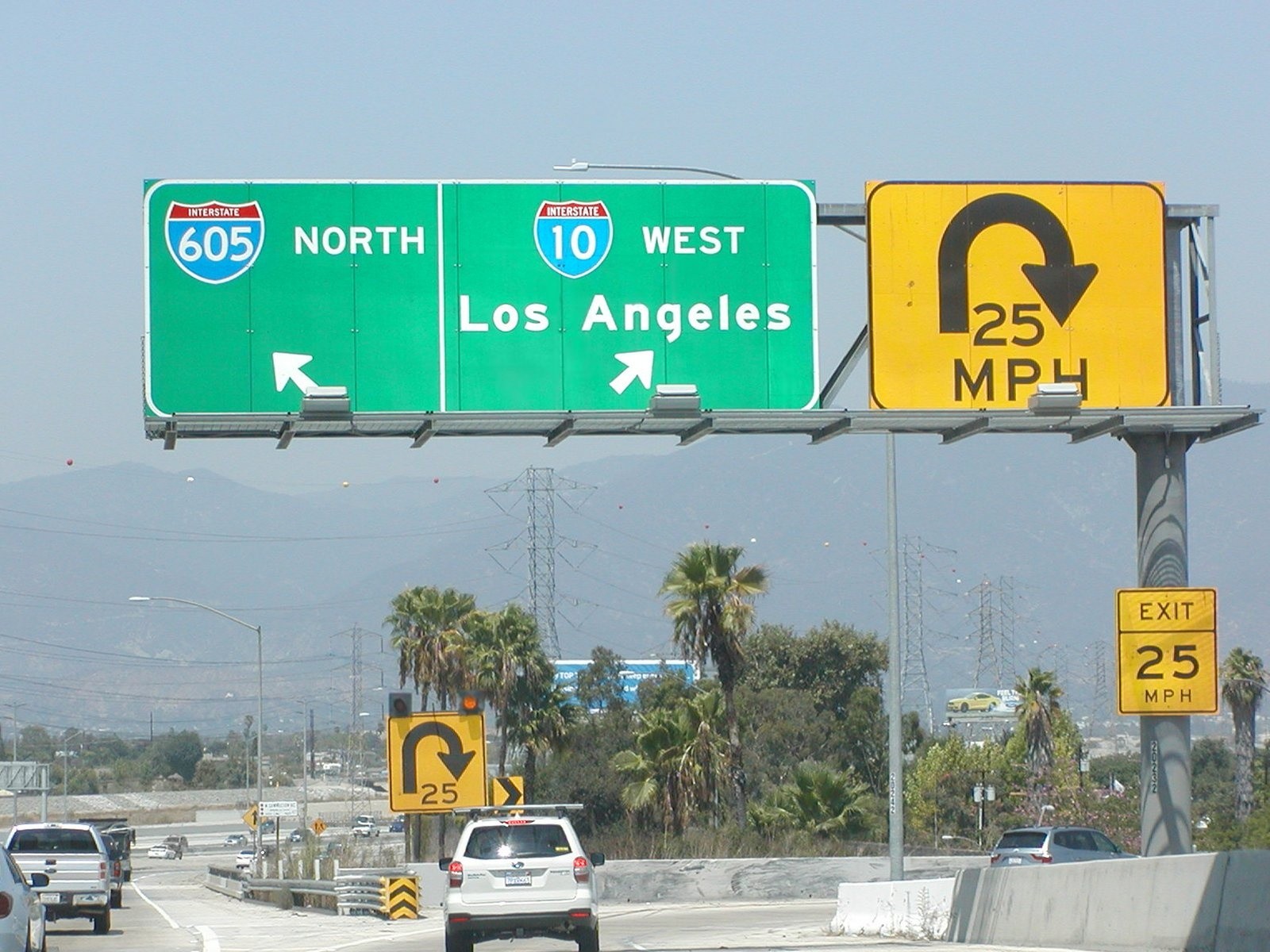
left=4, top=821, right=112, bottom=935
left=76, top=818, right=135, bottom=909
left=439, top=803, right=606, bottom=952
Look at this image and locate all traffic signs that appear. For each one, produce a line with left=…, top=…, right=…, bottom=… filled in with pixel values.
left=386, top=711, right=489, bottom=814
left=140, top=176, right=821, bottom=423
left=866, top=176, right=1172, bottom=413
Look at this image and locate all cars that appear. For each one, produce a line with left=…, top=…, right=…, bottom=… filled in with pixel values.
left=947, top=691, right=1002, bottom=714
left=235, top=848, right=258, bottom=870
left=288, top=827, right=317, bottom=843
left=248, top=818, right=276, bottom=835
left=988, top=826, right=1142, bottom=870
left=388, top=814, right=405, bottom=832
left=223, top=833, right=248, bottom=848
left=350, top=814, right=380, bottom=839
left=0, top=841, right=53, bottom=951
left=146, top=835, right=189, bottom=859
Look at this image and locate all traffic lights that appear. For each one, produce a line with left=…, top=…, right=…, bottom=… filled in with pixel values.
left=460, top=689, right=485, bottom=715
left=389, top=693, right=412, bottom=718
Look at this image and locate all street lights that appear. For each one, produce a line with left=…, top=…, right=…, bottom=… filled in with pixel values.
left=551, top=160, right=910, bottom=883
left=128, top=596, right=266, bottom=879
left=64, top=726, right=90, bottom=824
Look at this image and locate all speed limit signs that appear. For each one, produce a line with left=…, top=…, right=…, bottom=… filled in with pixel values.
left=1114, top=586, right=1219, bottom=716
